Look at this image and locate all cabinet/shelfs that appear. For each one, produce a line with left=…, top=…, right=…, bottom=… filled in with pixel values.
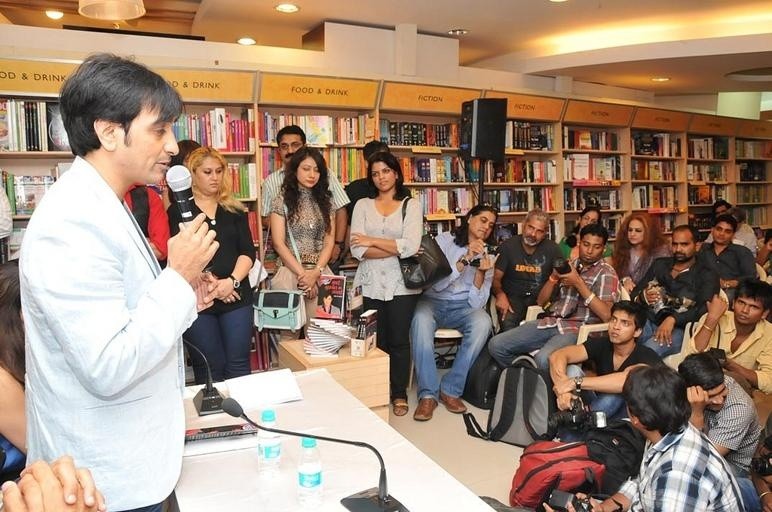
left=0, top=92, right=81, bottom=263
left=277, top=334, right=392, bottom=427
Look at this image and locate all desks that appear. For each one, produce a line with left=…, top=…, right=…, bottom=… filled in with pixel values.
left=168, top=366, right=502, bottom=512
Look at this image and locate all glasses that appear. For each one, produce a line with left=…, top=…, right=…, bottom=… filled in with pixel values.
left=280, top=142, right=303, bottom=149
left=477, top=202, right=499, bottom=212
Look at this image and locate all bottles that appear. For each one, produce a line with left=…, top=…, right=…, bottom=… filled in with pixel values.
left=297, top=437, right=324, bottom=510
left=255, top=410, right=282, bottom=477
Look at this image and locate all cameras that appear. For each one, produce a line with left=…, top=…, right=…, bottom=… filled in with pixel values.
left=651, top=289, right=679, bottom=325
left=549, top=488, right=593, bottom=511
left=749, top=435, right=770, bottom=476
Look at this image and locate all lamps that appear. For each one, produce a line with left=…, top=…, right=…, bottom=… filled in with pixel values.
left=75, top=0, right=148, bottom=22
left=715, top=92, right=772, bottom=122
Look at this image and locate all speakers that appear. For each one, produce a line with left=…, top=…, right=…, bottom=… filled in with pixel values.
left=460, top=98, right=510, bottom=158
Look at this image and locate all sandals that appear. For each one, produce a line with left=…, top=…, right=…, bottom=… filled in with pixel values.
left=391, top=394, right=408, bottom=416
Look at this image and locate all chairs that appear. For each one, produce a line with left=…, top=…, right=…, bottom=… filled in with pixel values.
left=519, top=305, right=611, bottom=372
left=662, top=319, right=699, bottom=374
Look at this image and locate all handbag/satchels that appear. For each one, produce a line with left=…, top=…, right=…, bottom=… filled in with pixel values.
left=460, top=344, right=504, bottom=410
left=252, top=290, right=307, bottom=334
left=397, top=197, right=452, bottom=289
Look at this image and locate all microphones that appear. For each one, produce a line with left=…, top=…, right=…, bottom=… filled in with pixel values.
left=183, top=338, right=258, bottom=441
left=165, top=164, right=214, bottom=273
left=222, top=398, right=410, bottom=512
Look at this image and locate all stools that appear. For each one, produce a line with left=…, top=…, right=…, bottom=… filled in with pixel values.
left=405, top=317, right=463, bottom=399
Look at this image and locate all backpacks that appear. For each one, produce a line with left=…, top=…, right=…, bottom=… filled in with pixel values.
left=464, top=354, right=558, bottom=447
left=581, top=416, right=646, bottom=497
left=509, top=440, right=606, bottom=512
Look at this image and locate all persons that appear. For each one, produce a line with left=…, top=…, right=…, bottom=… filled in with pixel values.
left=408, top=200, right=772, bottom=512
left=0, top=454, right=107, bottom=512
left=0, top=258, right=25, bottom=483
left=344, top=138, right=425, bottom=417
left=17, top=51, right=222, bottom=512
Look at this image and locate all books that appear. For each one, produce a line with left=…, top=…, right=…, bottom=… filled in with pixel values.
left=380, top=114, right=481, bottom=240
left=1, top=98, right=48, bottom=153
left=258, top=111, right=378, bottom=321
left=735, top=139, right=771, bottom=230
left=561, top=126, right=625, bottom=259
left=687, top=137, right=730, bottom=242
left=248, top=212, right=260, bottom=290
left=2, top=163, right=73, bottom=263
left=185, top=424, right=258, bottom=442
left=155, top=160, right=259, bottom=200
left=483, top=120, right=560, bottom=246
left=630, top=132, right=682, bottom=233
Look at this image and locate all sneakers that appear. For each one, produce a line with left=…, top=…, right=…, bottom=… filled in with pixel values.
left=439, top=388, right=468, bottom=413
left=414, top=397, right=438, bottom=421
